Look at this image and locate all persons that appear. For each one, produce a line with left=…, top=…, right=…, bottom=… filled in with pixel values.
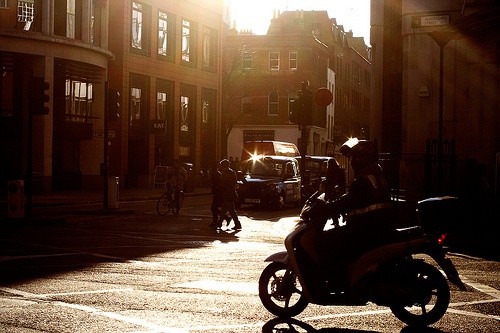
left=166, top=156, right=243, bottom=230
left=305, top=137, right=390, bottom=305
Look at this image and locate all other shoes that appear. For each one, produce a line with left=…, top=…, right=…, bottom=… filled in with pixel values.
left=231, top=224, right=241, bottom=229
left=227, top=218, right=231, bottom=227
left=209, top=223, right=216, bottom=226
left=213, top=222, right=222, bottom=227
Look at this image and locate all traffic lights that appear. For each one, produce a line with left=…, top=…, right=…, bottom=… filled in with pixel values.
left=288, top=97, right=303, bottom=124
left=107, top=89, right=120, bottom=120
left=32, top=77, right=49, bottom=116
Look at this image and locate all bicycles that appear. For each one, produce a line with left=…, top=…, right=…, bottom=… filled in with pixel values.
left=157, top=184, right=184, bottom=216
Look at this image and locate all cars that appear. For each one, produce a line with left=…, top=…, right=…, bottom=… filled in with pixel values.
left=294, top=156, right=346, bottom=205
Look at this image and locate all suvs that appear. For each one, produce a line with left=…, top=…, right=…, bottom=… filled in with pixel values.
left=234, top=156, right=301, bottom=211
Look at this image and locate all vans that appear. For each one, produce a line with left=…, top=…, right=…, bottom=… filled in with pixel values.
left=241, top=140, right=301, bottom=173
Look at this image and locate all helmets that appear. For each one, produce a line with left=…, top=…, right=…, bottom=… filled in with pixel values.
left=340, top=139, right=379, bottom=171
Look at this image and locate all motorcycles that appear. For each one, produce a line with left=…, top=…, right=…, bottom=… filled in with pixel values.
left=258, top=191, right=467, bottom=328
